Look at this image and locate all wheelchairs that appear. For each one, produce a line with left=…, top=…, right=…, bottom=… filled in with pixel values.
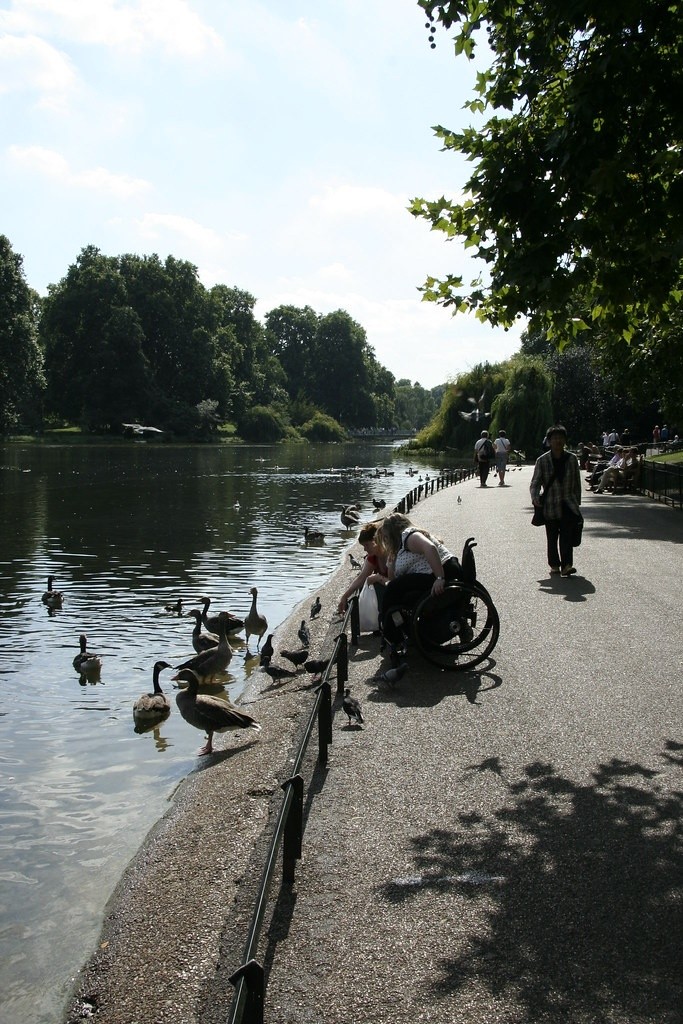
left=379, top=579, right=500, bottom=671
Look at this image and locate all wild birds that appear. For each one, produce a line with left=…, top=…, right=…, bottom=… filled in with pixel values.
left=457, top=496, right=462, bottom=503
left=512, top=468, right=516, bottom=472
left=326, top=465, right=465, bottom=481
left=348, top=554, right=361, bottom=569
left=258, top=595, right=336, bottom=685
left=494, top=468, right=510, bottom=477
left=518, top=468, right=522, bottom=471
left=342, top=688, right=364, bottom=726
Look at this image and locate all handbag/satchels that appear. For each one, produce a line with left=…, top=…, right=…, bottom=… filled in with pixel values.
left=474, top=453, right=478, bottom=462
left=358, top=577, right=379, bottom=631
left=532, top=495, right=544, bottom=526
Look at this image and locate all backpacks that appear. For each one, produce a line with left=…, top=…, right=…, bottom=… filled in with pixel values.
left=484, top=440, right=495, bottom=456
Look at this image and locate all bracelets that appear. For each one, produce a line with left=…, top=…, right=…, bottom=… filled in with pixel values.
left=436, top=576, right=444, bottom=580
left=380, top=575, right=391, bottom=587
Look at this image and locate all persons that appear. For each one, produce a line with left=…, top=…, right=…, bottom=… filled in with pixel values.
left=653, top=425, right=679, bottom=453
left=366, top=513, right=463, bottom=687
left=492, top=430, right=511, bottom=486
left=338, top=525, right=394, bottom=637
left=579, top=429, right=638, bottom=495
left=474, top=430, right=493, bottom=487
left=530, top=425, right=582, bottom=576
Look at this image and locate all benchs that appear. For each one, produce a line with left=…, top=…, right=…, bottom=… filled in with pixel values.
left=587, top=447, right=607, bottom=472
left=612, top=454, right=643, bottom=496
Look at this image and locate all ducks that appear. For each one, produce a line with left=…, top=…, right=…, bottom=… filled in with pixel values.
left=132, top=661, right=174, bottom=719
left=165, top=587, right=268, bottom=760
left=40, top=575, right=65, bottom=603
left=302, top=525, right=325, bottom=540
left=372, top=498, right=386, bottom=509
left=340, top=501, right=365, bottom=530
left=72, top=634, right=105, bottom=669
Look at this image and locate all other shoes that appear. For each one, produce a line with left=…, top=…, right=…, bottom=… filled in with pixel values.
left=585, top=479, right=594, bottom=486
left=586, top=475, right=592, bottom=478
left=562, top=564, right=576, bottom=576
left=551, top=566, right=560, bottom=574
left=586, top=487, right=596, bottom=491
left=594, top=489, right=602, bottom=494
